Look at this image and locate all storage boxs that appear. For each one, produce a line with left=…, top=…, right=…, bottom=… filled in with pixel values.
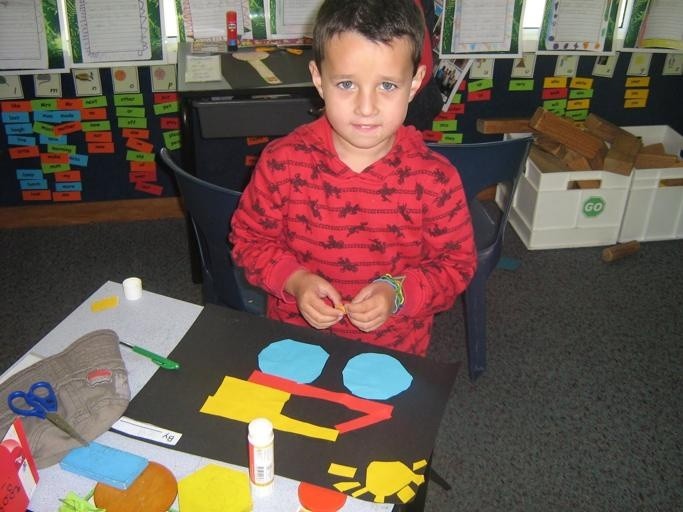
left=491, top=131, right=632, bottom=251
left=615, top=124, right=682, bottom=244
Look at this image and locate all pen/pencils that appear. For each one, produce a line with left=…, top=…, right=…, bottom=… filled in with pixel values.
left=116, top=337, right=182, bottom=371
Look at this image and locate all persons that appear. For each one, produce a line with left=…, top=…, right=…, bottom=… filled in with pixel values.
left=228, top=1, right=478, bottom=358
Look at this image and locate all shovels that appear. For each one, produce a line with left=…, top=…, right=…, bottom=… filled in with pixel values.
left=232, top=52, right=284, bottom=84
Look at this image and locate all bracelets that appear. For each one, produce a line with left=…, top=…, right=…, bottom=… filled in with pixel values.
left=374, top=273, right=407, bottom=313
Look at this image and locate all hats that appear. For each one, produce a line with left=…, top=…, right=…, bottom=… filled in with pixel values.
left=0, top=328, right=132, bottom=470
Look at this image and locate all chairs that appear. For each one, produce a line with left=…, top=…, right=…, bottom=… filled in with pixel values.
left=423, top=136, right=537, bottom=380
left=154, top=148, right=271, bottom=319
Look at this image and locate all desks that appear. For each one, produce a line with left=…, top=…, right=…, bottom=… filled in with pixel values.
left=171, top=35, right=324, bottom=290
left=0, top=279, right=466, bottom=511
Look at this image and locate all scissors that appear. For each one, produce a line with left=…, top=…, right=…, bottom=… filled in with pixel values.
left=8, top=382, right=89, bottom=447
